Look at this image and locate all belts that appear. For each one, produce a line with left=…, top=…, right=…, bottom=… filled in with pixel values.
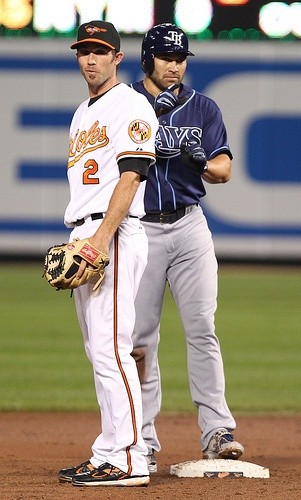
left=73, top=212, right=138, bottom=226
left=139, top=204, right=197, bottom=224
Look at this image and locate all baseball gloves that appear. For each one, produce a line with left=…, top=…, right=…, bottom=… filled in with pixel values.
left=42, top=238, right=110, bottom=298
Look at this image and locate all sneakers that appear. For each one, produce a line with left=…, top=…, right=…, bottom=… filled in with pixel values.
left=203, top=431, right=244, bottom=460
left=144, top=448, right=158, bottom=473
left=58, top=459, right=98, bottom=482
left=72, top=462, right=150, bottom=486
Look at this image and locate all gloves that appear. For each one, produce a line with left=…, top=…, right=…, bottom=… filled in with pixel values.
left=179, top=140, right=208, bottom=176
left=153, top=83, right=187, bottom=118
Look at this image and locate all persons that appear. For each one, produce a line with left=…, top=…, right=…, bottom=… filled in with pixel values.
left=42, top=20, right=159, bottom=487
left=126, top=23, right=245, bottom=473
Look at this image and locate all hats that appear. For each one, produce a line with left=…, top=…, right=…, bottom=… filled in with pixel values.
left=70, top=20, right=121, bottom=52
left=141, top=24, right=195, bottom=59
